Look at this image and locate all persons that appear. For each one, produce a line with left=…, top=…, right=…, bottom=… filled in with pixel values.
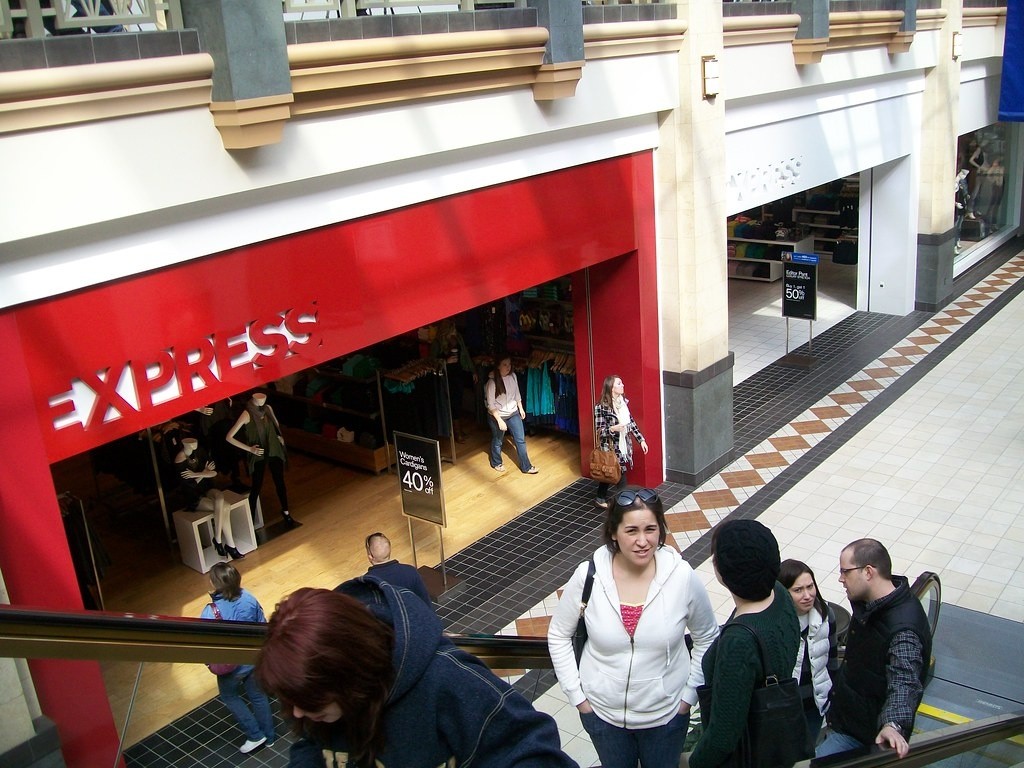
left=152, top=397, right=245, bottom=560
left=590, top=374, right=649, bottom=509
left=361, top=532, right=430, bottom=606
left=687, top=519, right=821, bottom=768
left=774, top=560, right=837, bottom=747
left=547, top=483, right=721, bottom=768
left=427, top=316, right=477, bottom=444
left=200, top=562, right=276, bottom=755
left=810, top=539, right=936, bottom=760
left=954, top=168, right=969, bottom=255
left=226, top=392, right=298, bottom=532
left=969, top=146, right=1006, bottom=234
left=255, top=574, right=578, bottom=768
left=482, top=352, right=540, bottom=474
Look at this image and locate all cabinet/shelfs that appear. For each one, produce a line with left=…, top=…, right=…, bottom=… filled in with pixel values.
left=727, top=210, right=860, bottom=282
left=276, top=372, right=394, bottom=475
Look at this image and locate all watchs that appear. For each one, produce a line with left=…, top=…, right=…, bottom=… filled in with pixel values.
left=884, top=720, right=902, bottom=734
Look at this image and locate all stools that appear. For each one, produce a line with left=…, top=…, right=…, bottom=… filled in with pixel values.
left=172, top=490, right=258, bottom=574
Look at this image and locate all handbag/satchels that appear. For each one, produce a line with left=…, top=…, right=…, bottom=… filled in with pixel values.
left=589, top=427, right=622, bottom=484
left=696, top=622, right=815, bottom=766
left=205, top=603, right=237, bottom=675
left=572, top=616, right=587, bottom=671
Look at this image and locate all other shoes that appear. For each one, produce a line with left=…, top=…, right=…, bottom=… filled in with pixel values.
left=282, top=511, right=294, bottom=524
left=265, top=742, right=275, bottom=747
left=594, top=501, right=609, bottom=509
left=494, top=464, right=506, bottom=472
left=239, top=736, right=267, bottom=753
left=526, top=466, right=540, bottom=473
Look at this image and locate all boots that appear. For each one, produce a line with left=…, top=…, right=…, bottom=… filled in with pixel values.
left=453, top=418, right=470, bottom=444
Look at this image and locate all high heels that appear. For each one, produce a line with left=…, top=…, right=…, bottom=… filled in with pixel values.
left=212, top=537, right=226, bottom=556
left=223, top=544, right=245, bottom=561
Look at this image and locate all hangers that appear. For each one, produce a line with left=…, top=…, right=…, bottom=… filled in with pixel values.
left=382, top=358, right=436, bottom=383
left=472, top=345, right=577, bottom=377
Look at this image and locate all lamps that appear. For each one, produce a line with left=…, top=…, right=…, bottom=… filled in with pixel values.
left=701, top=56, right=719, bottom=100
left=952, top=31, right=963, bottom=60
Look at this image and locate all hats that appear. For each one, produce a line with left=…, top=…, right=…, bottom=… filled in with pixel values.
left=716, top=519, right=780, bottom=601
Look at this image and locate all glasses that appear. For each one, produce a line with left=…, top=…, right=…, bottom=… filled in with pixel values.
left=611, top=487, right=659, bottom=510
left=839, top=564, right=876, bottom=574
